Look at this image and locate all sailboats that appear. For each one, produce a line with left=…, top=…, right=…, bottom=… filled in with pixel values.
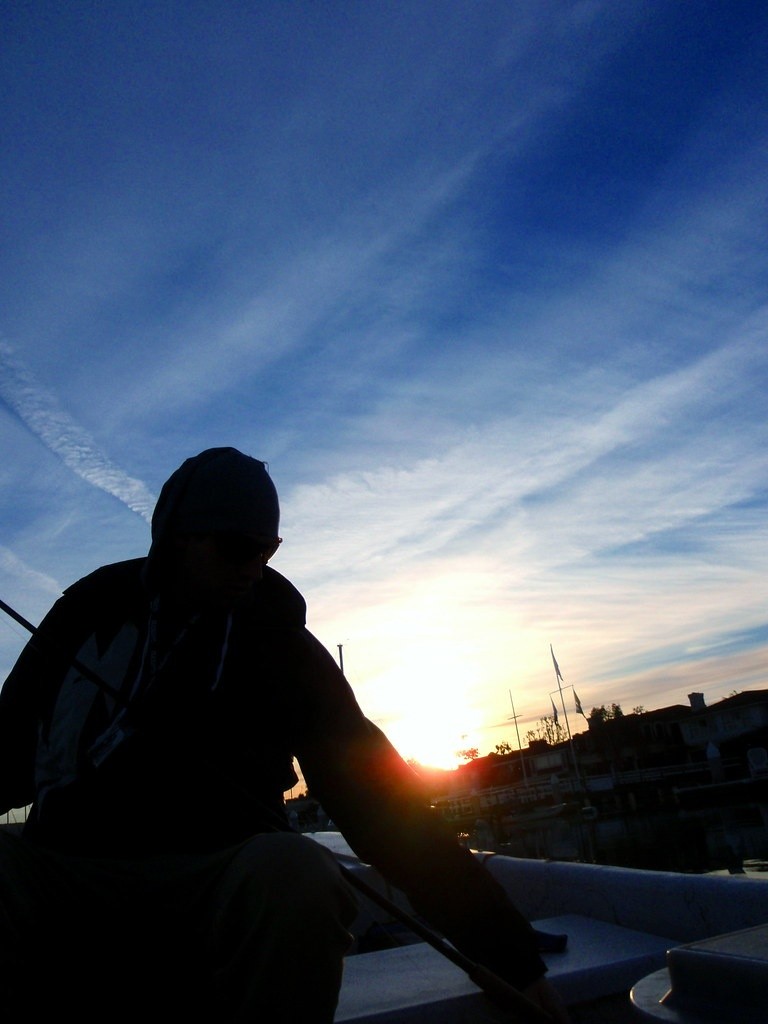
left=492, top=720, right=568, bottom=825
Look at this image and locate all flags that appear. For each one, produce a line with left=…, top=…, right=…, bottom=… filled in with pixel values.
left=551, top=699, right=558, bottom=723
left=574, top=690, right=583, bottom=714
left=551, top=649, right=564, bottom=682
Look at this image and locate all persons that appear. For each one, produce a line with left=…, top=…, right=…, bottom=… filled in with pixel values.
left=0, top=447, right=548, bottom=1024
